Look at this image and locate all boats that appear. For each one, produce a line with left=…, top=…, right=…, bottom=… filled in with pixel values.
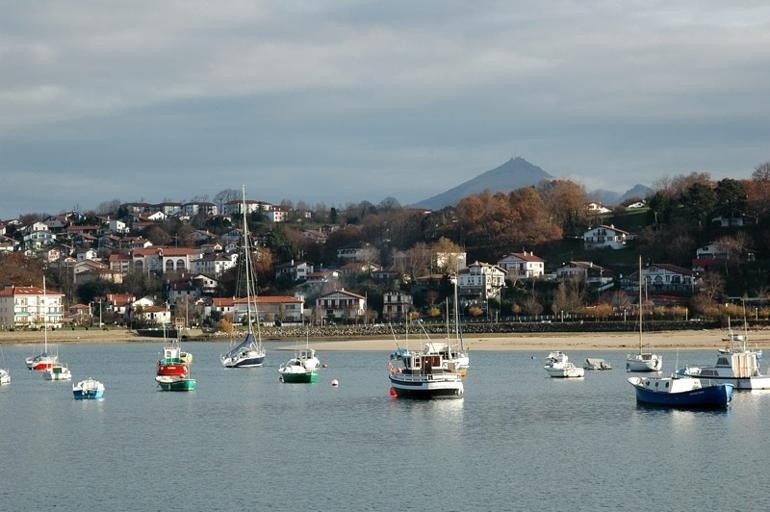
left=543, top=350, right=611, bottom=378
left=0, top=364, right=105, bottom=402
left=384, top=268, right=470, bottom=397
left=152, top=322, right=198, bottom=393
left=277, top=320, right=321, bottom=384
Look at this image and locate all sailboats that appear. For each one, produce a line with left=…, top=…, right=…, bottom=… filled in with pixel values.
left=23, top=274, right=60, bottom=370
left=624, top=253, right=770, bottom=407
left=215, top=184, right=268, bottom=369
